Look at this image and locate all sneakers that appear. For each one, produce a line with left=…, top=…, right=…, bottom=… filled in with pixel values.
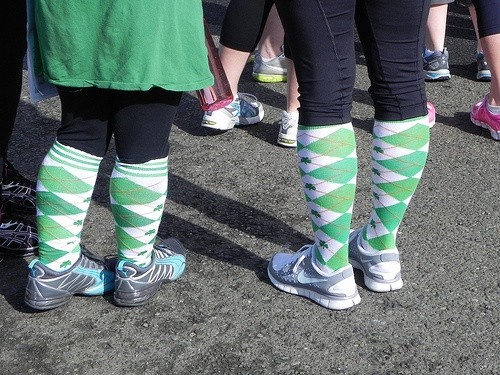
left=348, top=228, right=404, bottom=292
left=476, top=53, right=491, bottom=80
left=426, top=102, right=436, bottom=126
left=0, top=215, right=38, bottom=251
left=266, top=244, right=362, bottom=310
left=25, top=245, right=118, bottom=310
left=251, top=53, right=288, bottom=83
left=113, top=237, right=187, bottom=307
left=277, top=110, right=299, bottom=147
left=0, top=160, right=39, bottom=210
left=421, top=48, right=451, bottom=80
left=470, top=92, right=500, bottom=141
left=200, top=92, right=264, bottom=131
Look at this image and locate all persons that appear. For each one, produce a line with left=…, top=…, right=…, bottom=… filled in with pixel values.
left=195, top=0, right=500, bottom=149
left=26, top=0, right=215, bottom=310
left=1, top=0, right=38, bottom=255
left=267, top=0, right=430, bottom=310
